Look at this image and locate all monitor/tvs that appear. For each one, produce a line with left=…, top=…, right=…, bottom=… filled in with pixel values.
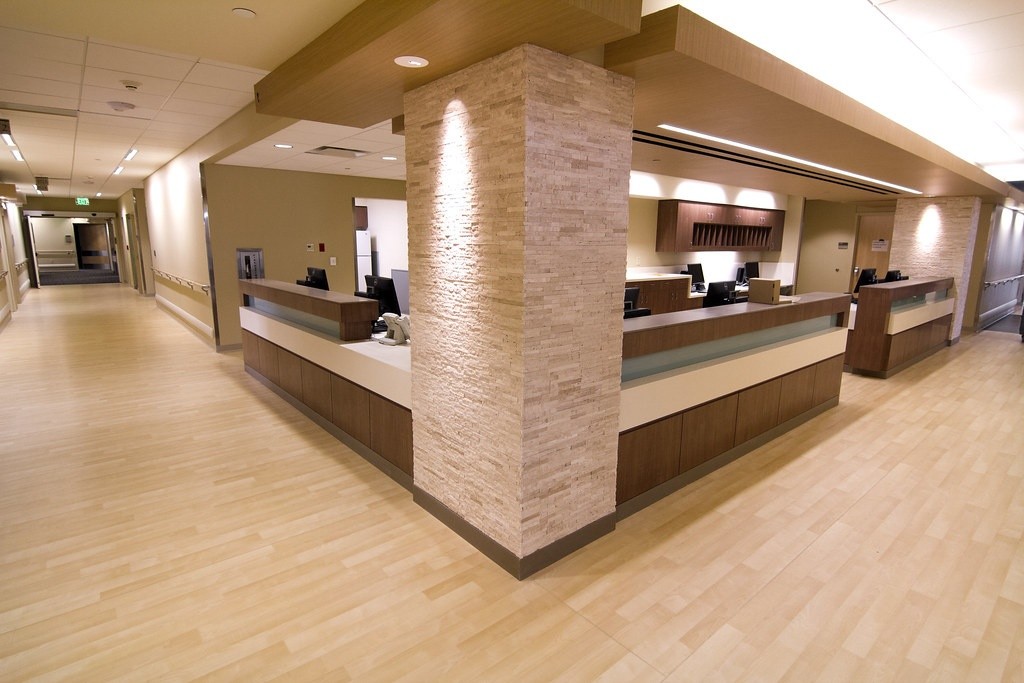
left=687, top=263, right=705, bottom=292
left=745, top=262, right=759, bottom=280
left=884, top=270, right=902, bottom=283
left=703, top=281, right=739, bottom=307
left=305, top=267, right=329, bottom=290
left=854, top=268, right=877, bottom=293
left=365, top=275, right=401, bottom=327
left=624, top=287, right=640, bottom=311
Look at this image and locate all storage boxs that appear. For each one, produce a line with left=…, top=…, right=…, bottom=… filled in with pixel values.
left=748, top=277, right=781, bottom=305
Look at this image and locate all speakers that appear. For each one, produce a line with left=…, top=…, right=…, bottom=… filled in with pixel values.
left=736, top=268, right=744, bottom=282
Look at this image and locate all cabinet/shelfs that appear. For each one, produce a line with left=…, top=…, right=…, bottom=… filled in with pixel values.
left=655, top=200, right=786, bottom=252
